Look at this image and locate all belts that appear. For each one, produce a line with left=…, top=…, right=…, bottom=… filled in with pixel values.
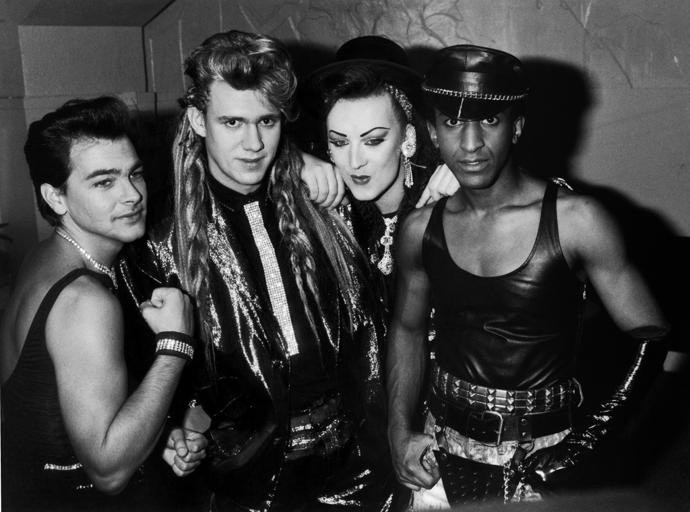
left=423, top=384, right=582, bottom=445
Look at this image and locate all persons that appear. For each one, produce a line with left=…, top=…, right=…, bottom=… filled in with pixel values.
left=382, top=46, right=671, bottom=509
left=287, top=35, right=443, bottom=355
left=1, top=97, right=209, bottom=512
left=156, top=30, right=393, bottom=507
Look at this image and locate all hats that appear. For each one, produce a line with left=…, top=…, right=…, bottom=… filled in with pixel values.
left=309, top=35, right=426, bottom=90
left=417, top=43, right=530, bottom=124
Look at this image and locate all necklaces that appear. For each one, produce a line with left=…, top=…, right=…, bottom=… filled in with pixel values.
left=54, top=227, right=144, bottom=312
left=373, top=212, right=401, bottom=276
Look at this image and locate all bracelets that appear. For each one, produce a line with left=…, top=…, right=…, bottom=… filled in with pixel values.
left=152, top=330, right=197, bottom=362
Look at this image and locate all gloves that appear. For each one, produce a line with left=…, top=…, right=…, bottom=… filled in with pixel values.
left=521, top=324, right=682, bottom=493
